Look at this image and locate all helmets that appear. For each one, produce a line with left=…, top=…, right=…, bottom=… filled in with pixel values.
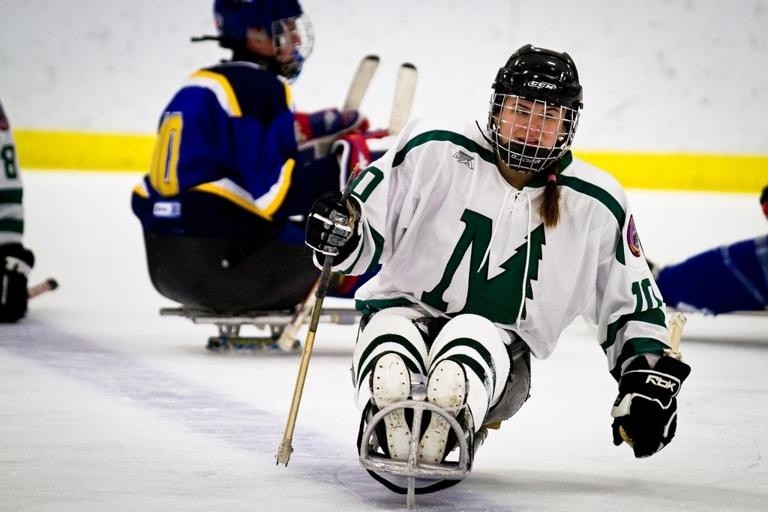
left=211, top=0, right=305, bottom=52
left=489, top=43, right=585, bottom=156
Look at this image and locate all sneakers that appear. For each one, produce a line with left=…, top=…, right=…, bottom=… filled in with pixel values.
left=367, top=349, right=418, bottom=466
left=414, top=356, right=468, bottom=466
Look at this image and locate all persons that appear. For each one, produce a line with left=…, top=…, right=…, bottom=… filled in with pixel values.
left=301, top=41, right=689, bottom=497
left=0, top=102, right=34, bottom=325
left=642, top=183, right=767, bottom=317
left=130, top=0, right=385, bottom=314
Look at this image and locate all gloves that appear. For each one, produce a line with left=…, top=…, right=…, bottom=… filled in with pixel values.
left=609, top=353, right=694, bottom=459
left=302, top=189, right=364, bottom=257
left=294, top=106, right=358, bottom=157
left=1, top=243, right=36, bottom=326
left=329, top=120, right=399, bottom=197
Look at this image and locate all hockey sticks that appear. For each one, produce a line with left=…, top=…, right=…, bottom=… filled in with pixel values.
left=365, top=64, right=418, bottom=139
left=298, top=54, right=380, bottom=148
